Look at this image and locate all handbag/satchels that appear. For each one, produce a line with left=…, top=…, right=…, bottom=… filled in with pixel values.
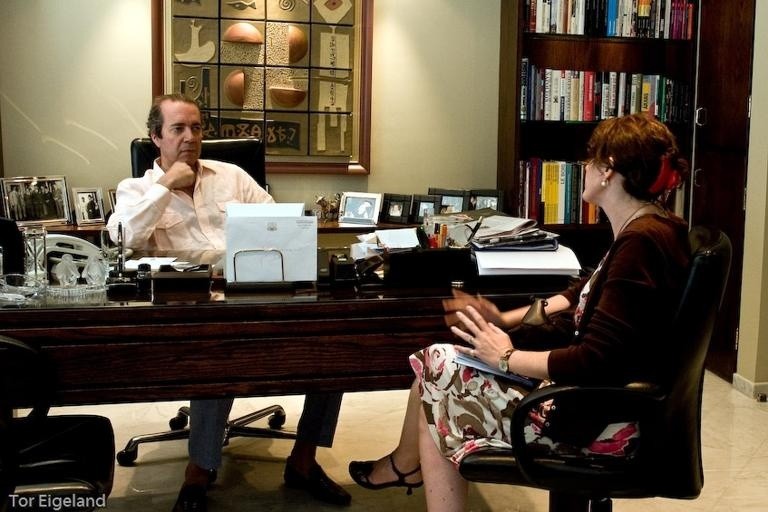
left=506, top=296, right=578, bottom=349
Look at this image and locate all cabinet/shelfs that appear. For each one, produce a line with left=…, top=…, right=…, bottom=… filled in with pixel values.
left=494, top=0, right=711, bottom=268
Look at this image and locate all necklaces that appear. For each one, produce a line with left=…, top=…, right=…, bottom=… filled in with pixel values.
left=618, top=203, right=649, bottom=234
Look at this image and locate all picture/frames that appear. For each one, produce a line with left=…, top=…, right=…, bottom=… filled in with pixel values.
left=72, top=188, right=107, bottom=227
left=0, top=174, right=74, bottom=228
left=338, top=187, right=503, bottom=230
left=151, top=1, right=375, bottom=177
left=106, top=189, right=117, bottom=213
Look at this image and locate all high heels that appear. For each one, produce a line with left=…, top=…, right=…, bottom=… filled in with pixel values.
left=349, top=454, right=423, bottom=495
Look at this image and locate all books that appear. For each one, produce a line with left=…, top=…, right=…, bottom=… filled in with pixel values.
left=520, top=0, right=694, bottom=222
left=452, top=351, right=534, bottom=388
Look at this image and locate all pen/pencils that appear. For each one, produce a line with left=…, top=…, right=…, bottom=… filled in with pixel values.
left=464, top=216, right=483, bottom=246
left=416, top=223, right=447, bottom=249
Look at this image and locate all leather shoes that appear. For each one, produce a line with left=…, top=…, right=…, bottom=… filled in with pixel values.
left=284, top=456, right=352, bottom=506
left=172, top=482, right=206, bottom=512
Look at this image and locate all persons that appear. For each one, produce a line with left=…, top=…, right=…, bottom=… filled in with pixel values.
left=107, top=92, right=352, bottom=512
left=349, top=114, right=694, bottom=511
left=81, top=195, right=96, bottom=220
left=390, top=203, right=401, bottom=216
left=9, top=184, right=58, bottom=220
left=356, top=200, right=372, bottom=219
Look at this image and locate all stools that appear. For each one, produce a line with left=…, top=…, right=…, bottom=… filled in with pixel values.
left=0, top=335, right=115, bottom=512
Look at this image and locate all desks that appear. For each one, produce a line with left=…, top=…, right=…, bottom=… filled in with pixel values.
left=1, top=262, right=562, bottom=413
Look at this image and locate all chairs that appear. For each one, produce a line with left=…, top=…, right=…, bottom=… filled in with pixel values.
left=118, top=133, right=303, bottom=466
left=456, top=214, right=732, bottom=510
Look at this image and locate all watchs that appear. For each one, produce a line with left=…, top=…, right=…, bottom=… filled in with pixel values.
left=499, top=348, right=517, bottom=375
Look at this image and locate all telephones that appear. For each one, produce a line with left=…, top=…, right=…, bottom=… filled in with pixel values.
left=28, top=233, right=109, bottom=267
